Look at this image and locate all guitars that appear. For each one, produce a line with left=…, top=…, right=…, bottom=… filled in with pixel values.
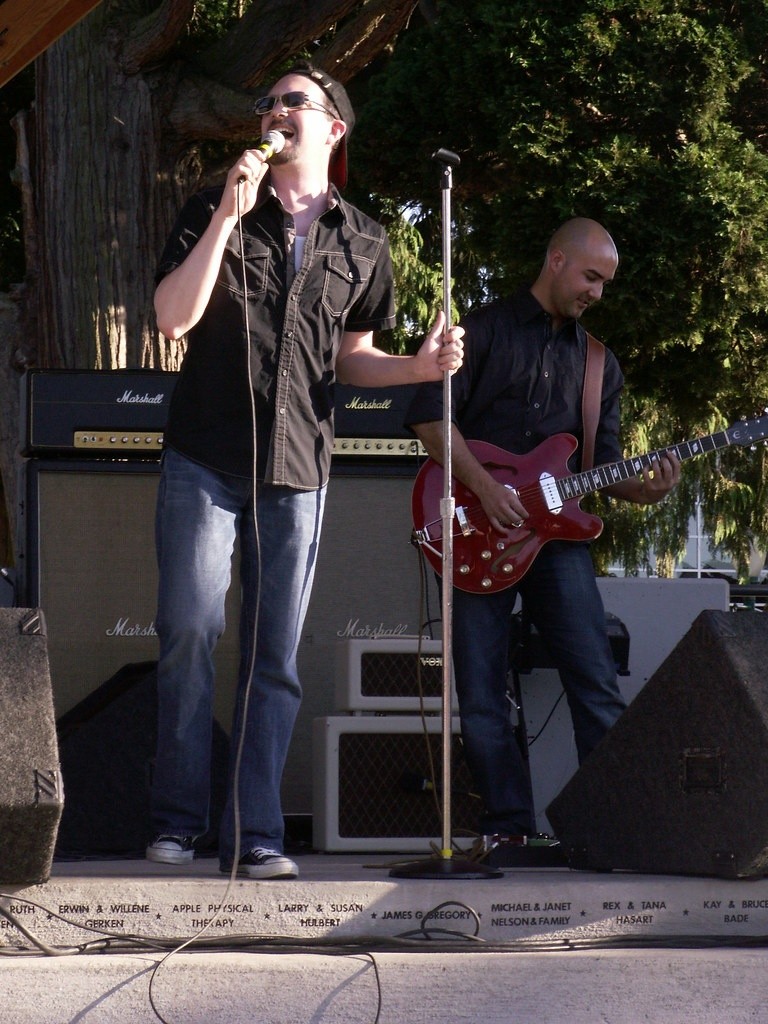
left=411, top=401, right=768, bottom=596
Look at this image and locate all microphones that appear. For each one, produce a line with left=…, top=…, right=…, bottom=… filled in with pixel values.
left=238, top=129, right=286, bottom=183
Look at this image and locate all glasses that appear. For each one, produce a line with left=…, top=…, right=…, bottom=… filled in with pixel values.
left=252, top=91, right=338, bottom=120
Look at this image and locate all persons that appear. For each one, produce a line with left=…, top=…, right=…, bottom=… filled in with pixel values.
left=145, top=62, right=467, bottom=880
left=403, top=216, right=682, bottom=872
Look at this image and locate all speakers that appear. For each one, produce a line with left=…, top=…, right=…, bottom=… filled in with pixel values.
left=495, top=574, right=768, bottom=882
left=19, top=460, right=444, bottom=856
left=0, top=608, right=66, bottom=883
left=313, top=715, right=492, bottom=856
left=335, top=639, right=460, bottom=714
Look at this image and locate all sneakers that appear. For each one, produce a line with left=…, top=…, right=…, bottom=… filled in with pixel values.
left=221, top=846, right=299, bottom=880
left=145, top=835, right=194, bottom=864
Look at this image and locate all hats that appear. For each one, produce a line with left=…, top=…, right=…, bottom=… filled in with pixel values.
left=287, top=67, right=356, bottom=189
